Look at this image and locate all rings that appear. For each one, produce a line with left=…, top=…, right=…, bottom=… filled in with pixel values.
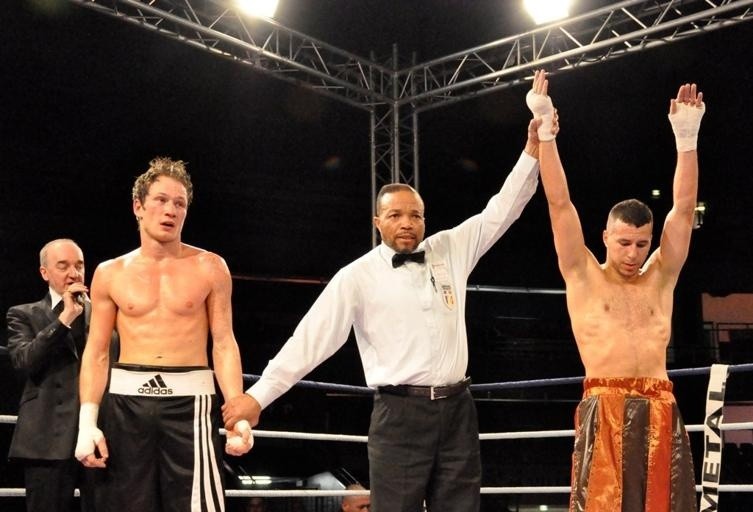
left=68, top=289, right=74, bottom=296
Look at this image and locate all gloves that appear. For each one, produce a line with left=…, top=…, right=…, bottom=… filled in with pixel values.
left=526, top=89, right=557, bottom=142
left=668, top=101, right=706, bottom=153
left=75, top=402, right=103, bottom=460
left=227, top=421, right=254, bottom=448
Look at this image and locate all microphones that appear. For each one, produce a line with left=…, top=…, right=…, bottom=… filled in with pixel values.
left=73, top=291, right=84, bottom=305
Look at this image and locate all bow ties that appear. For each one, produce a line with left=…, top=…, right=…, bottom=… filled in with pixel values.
left=392, top=251, right=425, bottom=268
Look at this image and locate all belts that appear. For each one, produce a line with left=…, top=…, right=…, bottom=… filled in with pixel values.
left=378, top=376, right=472, bottom=400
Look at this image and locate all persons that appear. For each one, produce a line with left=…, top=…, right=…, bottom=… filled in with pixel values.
left=6, top=238, right=119, bottom=512
left=526, top=69, right=706, bottom=512
left=340, top=484, right=371, bottom=512
left=221, top=108, right=559, bottom=512
left=74, top=157, right=255, bottom=512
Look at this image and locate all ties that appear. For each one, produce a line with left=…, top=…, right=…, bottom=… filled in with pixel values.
left=72, top=304, right=86, bottom=362
